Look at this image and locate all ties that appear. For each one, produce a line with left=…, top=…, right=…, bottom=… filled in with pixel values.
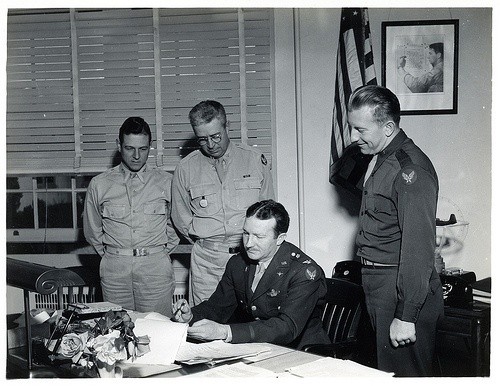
left=251, top=263, right=266, bottom=293
left=362, top=154, right=378, bottom=186
left=214, top=158, right=225, bottom=182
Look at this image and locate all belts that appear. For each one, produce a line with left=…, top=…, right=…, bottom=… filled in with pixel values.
left=200, top=242, right=242, bottom=254
left=106, top=246, right=165, bottom=256
left=361, top=258, right=394, bottom=267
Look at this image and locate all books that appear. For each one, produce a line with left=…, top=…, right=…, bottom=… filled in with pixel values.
left=62, top=301, right=123, bottom=324
left=464, top=277, right=491, bottom=305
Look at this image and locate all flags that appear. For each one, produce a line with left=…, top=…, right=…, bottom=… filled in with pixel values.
left=328, top=8, right=379, bottom=192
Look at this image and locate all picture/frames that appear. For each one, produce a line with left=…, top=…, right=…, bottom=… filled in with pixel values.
left=381, top=19, right=459, bottom=116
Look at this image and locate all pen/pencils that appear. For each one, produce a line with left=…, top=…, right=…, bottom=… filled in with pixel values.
left=173, top=299, right=187, bottom=318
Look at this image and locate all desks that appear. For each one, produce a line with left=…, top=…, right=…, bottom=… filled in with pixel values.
left=437, top=299, right=490, bottom=377
left=7, top=323, right=253, bottom=378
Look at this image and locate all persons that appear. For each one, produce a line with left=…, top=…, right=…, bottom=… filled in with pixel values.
left=171, top=100, right=275, bottom=307
left=84, top=116, right=181, bottom=319
left=397, top=42, right=444, bottom=94
left=347, top=84, right=444, bottom=377
left=173, top=200, right=336, bottom=358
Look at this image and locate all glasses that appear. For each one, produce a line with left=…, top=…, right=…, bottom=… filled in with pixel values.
left=196, top=124, right=226, bottom=146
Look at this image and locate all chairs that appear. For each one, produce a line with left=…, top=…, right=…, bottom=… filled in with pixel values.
left=305, top=279, right=370, bottom=364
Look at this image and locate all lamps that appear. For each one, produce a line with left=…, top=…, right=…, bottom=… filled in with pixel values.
left=6, top=257, right=85, bottom=378
